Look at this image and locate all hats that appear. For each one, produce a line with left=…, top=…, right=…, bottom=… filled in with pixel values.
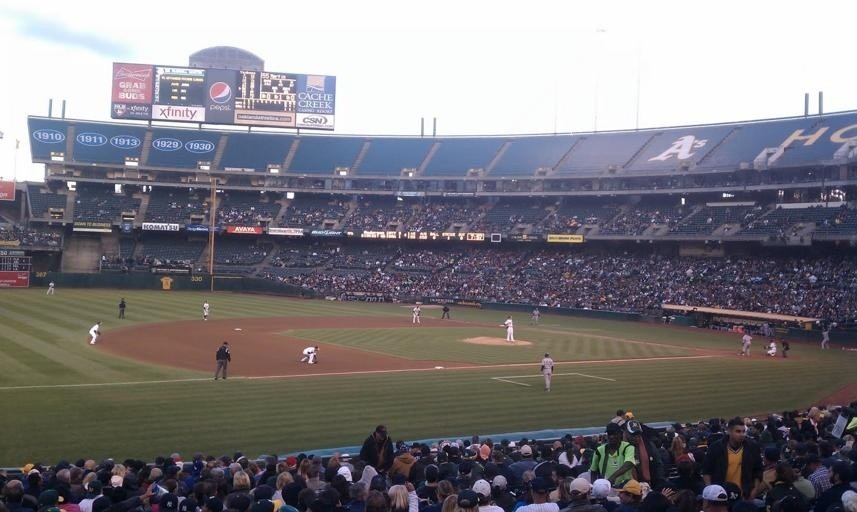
left=44, top=424, right=803, bottom=511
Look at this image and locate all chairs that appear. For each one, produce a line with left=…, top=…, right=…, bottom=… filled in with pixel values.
left=0, top=115, right=857, bottom=350
